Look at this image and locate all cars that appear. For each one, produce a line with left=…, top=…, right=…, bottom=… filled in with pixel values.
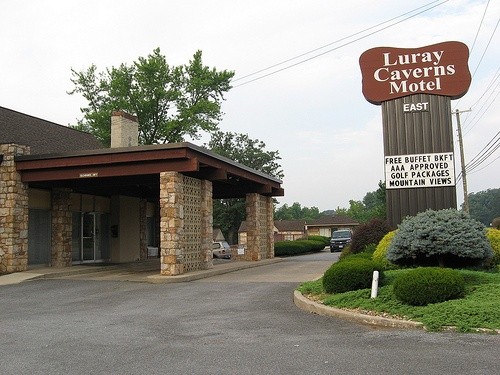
left=213, top=241, right=232, bottom=260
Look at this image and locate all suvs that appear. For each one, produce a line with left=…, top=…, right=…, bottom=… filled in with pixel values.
left=327, top=230, right=353, bottom=253
